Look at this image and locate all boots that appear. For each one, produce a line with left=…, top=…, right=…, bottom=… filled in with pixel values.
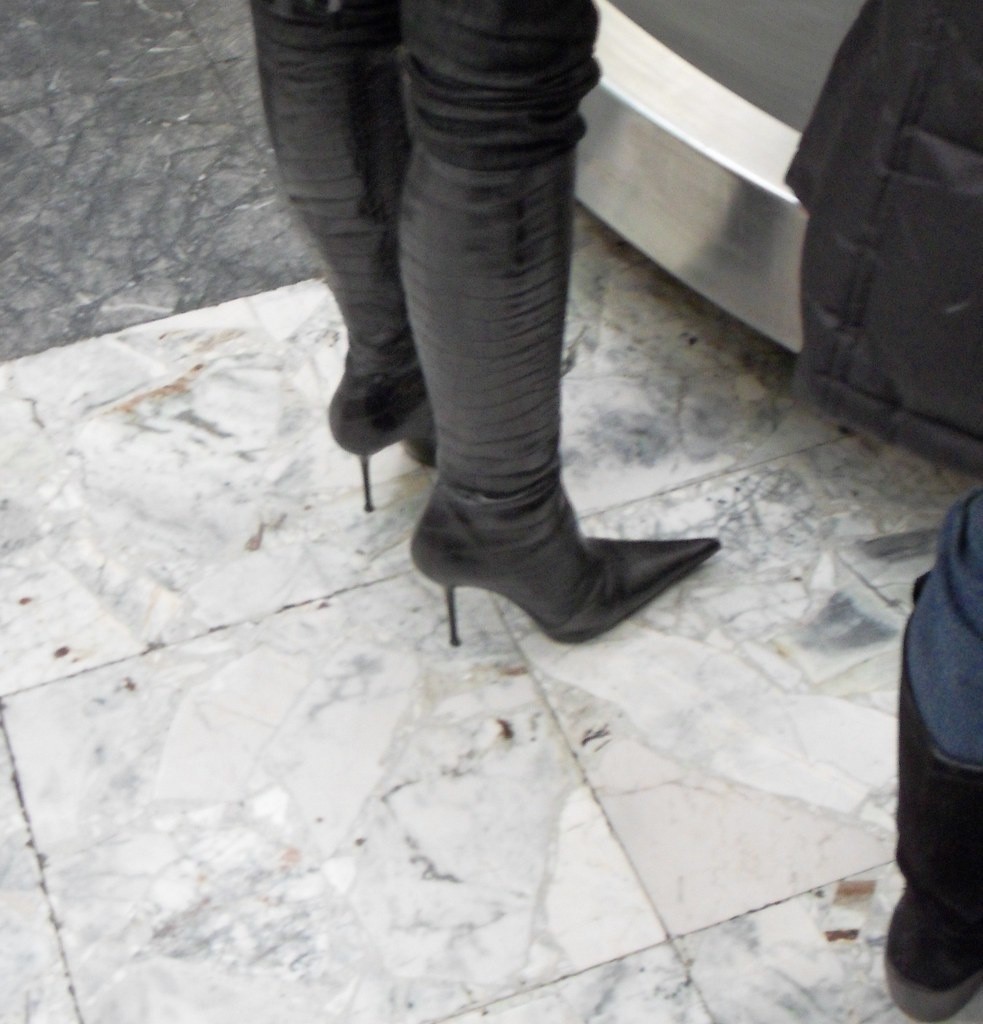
left=885, top=567, right=983, bottom=1022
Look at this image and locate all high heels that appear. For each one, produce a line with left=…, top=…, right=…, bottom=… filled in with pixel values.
left=330, top=352, right=437, bottom=513
left=407, top=482, right=721, bottom=647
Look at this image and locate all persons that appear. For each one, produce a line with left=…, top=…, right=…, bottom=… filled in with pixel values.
left=882, top=484, right=982, bottom=1023
left=250, top=0, right=720, bottom=646
